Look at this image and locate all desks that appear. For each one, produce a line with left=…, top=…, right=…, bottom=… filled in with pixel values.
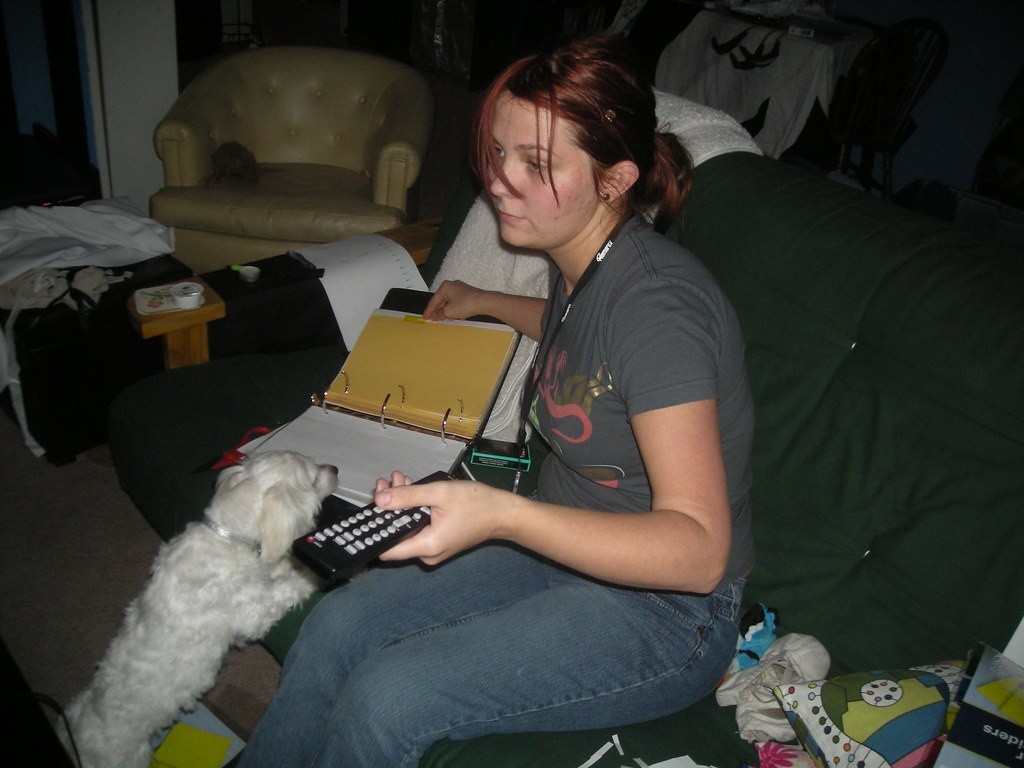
left=624, top=0, right=885, bottom=160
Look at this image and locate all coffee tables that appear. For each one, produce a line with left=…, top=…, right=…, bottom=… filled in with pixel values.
left=18, top=255, right=194, bottom=386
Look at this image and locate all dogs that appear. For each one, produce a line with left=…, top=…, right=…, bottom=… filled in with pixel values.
left=56, top=449, right=340, bottom=768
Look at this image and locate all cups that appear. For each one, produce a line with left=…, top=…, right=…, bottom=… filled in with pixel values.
left=239, top=266, right=260, bottom=282
left=171, top=281, right=204, bottom=308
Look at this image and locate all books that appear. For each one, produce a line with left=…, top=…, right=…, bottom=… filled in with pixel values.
left=238, top=288, right=524, bottom=511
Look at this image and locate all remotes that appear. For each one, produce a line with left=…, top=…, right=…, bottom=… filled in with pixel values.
left=292, top=471, right=463, bottom=585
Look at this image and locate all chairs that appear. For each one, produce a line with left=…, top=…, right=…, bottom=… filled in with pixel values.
left=828, top=15, right=950, bottom=203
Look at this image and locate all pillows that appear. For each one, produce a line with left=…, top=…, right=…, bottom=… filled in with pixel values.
left=772, top=657, right=966, bottom=768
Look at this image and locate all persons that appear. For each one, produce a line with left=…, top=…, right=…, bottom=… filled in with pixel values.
left=235, top=53, right=755, bottom=768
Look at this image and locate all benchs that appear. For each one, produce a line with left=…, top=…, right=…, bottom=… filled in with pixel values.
left=128, top=217, right=442, bottom=370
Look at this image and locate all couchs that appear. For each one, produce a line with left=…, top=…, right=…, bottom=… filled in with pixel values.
left=149, top=44, right=435, bottom=276
left=92, top=146, right=1024, bottom=768
